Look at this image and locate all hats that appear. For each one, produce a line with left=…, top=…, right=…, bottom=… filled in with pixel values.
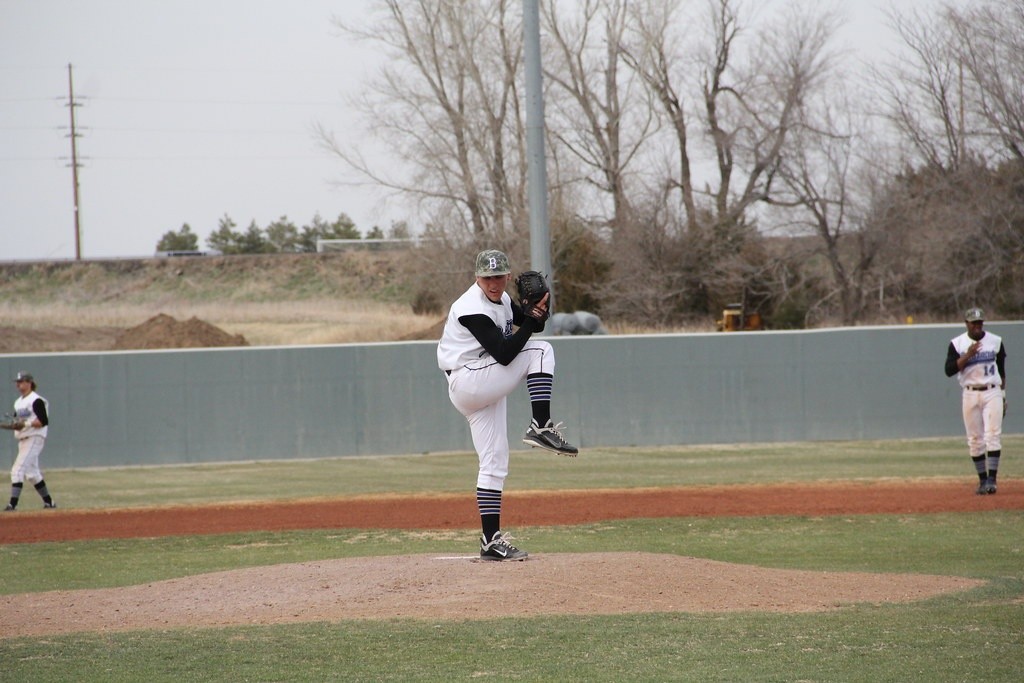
left=965, top=308, right=984, bottom=322
left=476, top=249, right=511, bottom=277
left=12, top=371, right=33, bottom=382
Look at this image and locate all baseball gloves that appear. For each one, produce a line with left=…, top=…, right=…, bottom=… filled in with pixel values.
left=0, top=412, right=27, bottom=431
left=514, top=269, right=551, bottom=323
left=1003, top=397, right=1007, bottom=419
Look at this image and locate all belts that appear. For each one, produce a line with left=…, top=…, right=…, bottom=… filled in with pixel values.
left=967, top=385, right=995, bottom=391
left=446, top=370, right=451, bottom=374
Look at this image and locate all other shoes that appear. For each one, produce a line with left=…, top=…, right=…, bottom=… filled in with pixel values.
left=43, top=501, right=56, bottom=510
left=4, top=504, right=17, bottom=511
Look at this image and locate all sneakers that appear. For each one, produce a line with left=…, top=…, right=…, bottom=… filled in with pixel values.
left=479, top=531, right=528, bottom=562
left=986, top=477, right=997, bottom=493
left=523, top=417, right=579, bottom=458
left=976, top=481, right=986, bottom=494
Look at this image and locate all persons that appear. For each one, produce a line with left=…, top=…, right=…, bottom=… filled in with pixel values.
left=1, top=372, right=57, bottom=511
left=437, top=249, right=578, bottom=562
left=945, top=309, right=1007, bottom=494
